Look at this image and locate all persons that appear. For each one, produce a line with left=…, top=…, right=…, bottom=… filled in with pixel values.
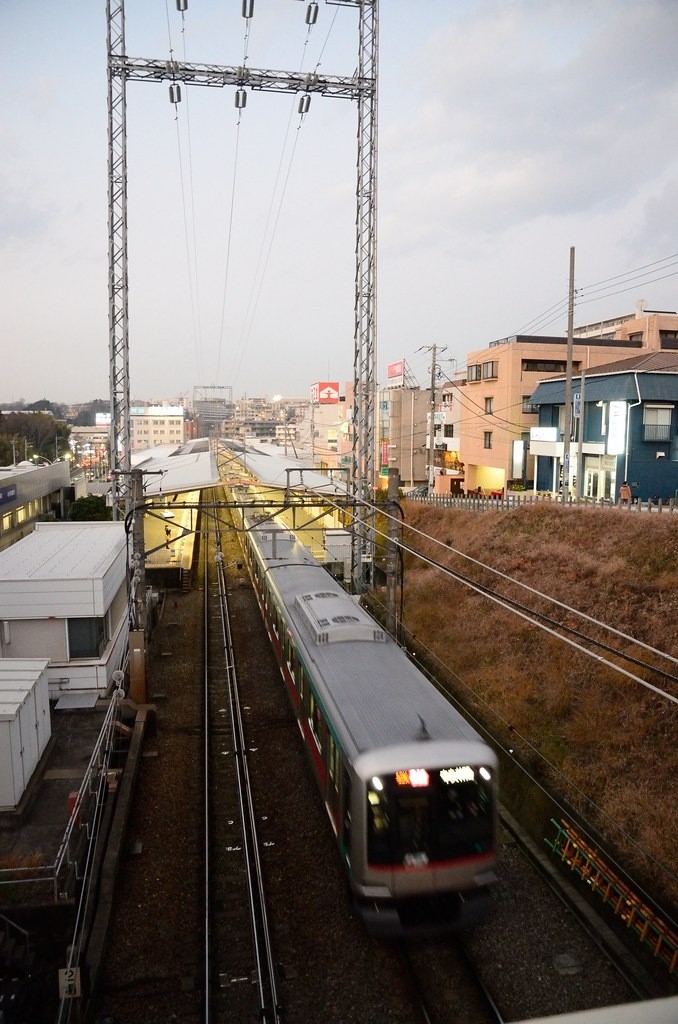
left=619, top=481, right=631, bottom=505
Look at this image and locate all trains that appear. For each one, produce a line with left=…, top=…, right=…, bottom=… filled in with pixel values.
left=216, top=451, right=499, bottom=939
